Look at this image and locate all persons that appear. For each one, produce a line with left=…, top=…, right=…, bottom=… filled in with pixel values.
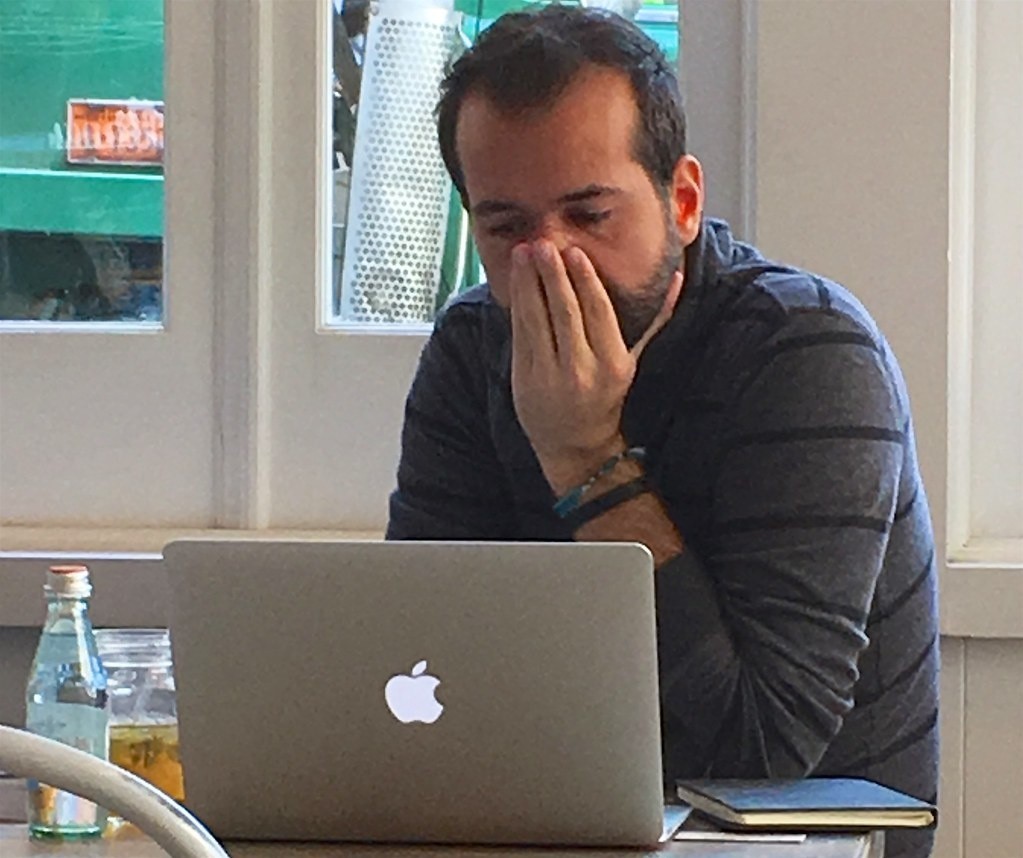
left=382, top=4, right=940, bottom=858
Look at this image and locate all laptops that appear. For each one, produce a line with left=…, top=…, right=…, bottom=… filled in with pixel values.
left=162, top=538, right=695, bottom=849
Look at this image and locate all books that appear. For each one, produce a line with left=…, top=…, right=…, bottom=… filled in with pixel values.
left=680, top=778, right=940, bottom=836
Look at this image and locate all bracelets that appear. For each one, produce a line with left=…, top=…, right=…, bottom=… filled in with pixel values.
left=559, top=475, right=654, bottom=538
left=550, top=445, right=647, bottom=514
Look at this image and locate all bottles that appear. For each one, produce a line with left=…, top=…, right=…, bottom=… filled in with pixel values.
left=27, top=283, right=161, bottom=321
left=24, top=565, right=111, bottom=841
left=88, top=626, right=185, bottom=841
